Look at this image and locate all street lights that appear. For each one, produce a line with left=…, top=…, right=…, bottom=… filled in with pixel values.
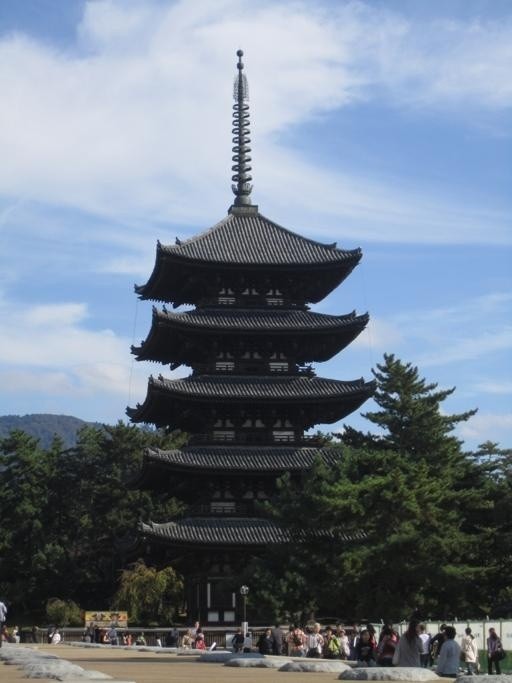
left=239, top=583, right=249, bottom=639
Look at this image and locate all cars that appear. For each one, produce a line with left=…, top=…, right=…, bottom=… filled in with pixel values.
left=321, top=627, right=359, bottom=645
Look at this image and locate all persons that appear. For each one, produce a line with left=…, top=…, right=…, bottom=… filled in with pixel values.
left=82, top=623, right=146, bottom=646
left=428, top=623, right=447, bottom=664
left=460, top=628, right=477, bottom=675
left=32, top=623, right=39, bottom=643
left=486, top=627, right=505, bottom=674
left=435, top=625, right=460, bottom=677
left=257, top=617, right=430, bottom=666
left=164, top=622, right=206, bottom=650
left=47, top=625, right=61, bottom=644
left=155, top=633, right=162, bottom=646
left=2, top=624, right=25, bottom=643
left=232, top=629, right=252, bottom=653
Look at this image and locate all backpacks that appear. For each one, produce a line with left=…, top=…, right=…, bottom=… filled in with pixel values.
left=327, top=641, right=339, bottom=657
left=111, top=639, right=117, bottom=645
left=184, top=639, right=190, bottom=645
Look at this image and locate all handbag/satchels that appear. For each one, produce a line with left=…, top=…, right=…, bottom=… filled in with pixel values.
left=495, top=647, right=506, bottom=660
left=293, top=636, right=302, bottom=646
left=48, top=637, right=52, bottom=644
left=460, top=651, right=466, bottom=662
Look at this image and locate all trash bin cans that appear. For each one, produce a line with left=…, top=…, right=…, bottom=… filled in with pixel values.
left=22, top=629, right=33, bottom=643
